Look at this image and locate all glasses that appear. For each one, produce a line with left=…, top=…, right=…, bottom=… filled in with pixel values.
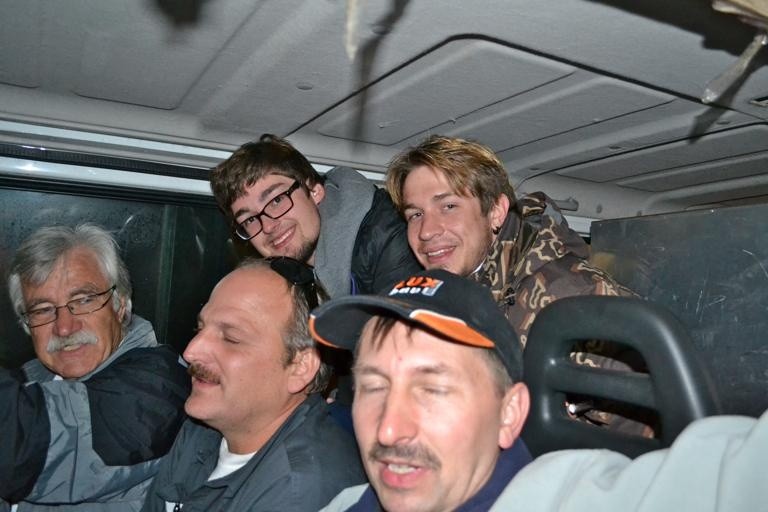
left=235, top=180, right=300, bottom=241
left=21, top=285, right=117, bottom=329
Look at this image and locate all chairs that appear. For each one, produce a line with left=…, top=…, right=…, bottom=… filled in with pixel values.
left=521, top=298, right=723, bottom=459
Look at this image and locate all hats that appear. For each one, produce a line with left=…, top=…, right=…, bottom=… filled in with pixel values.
left=308, top=268, right=525, bottom=350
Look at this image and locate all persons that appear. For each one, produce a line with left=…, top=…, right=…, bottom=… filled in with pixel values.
left=309, top=269, right=767, bottom=511
left=208, top=135, right=424, bottom=313
left=385, top=136, right=653, bottom=440
left=140, top=257, right=369, bottom=511
left=2, top=219, right=193, bottom=512
